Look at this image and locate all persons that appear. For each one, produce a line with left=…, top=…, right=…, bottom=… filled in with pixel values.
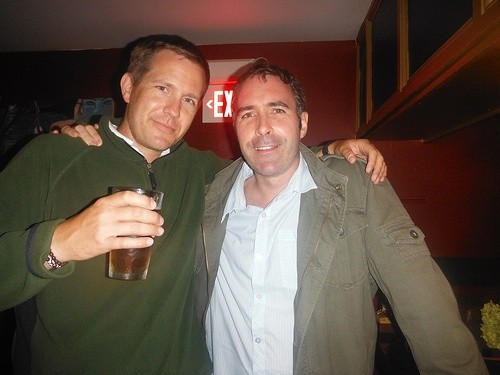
left=48, top=58, right=489, bottom=375
left=0, top=34, right=388, bottom=375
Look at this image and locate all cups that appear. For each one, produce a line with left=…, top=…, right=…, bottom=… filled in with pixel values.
left=108, top=186, right=163, bottom=281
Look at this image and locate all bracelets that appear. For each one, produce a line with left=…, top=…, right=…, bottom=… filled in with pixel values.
left=322, top=139, right=336, bottom=156
left=46, top=249, right=68, bottom=269
